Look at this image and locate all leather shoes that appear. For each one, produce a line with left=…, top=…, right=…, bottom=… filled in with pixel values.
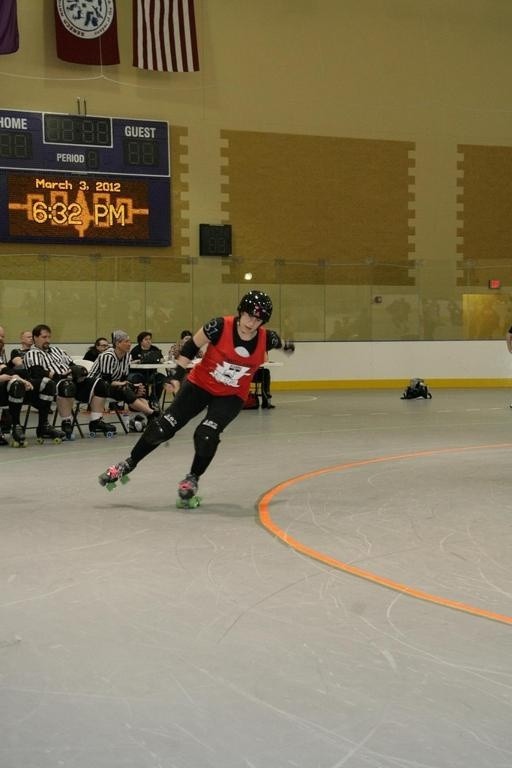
left=261, top=403, right=276, bottom=409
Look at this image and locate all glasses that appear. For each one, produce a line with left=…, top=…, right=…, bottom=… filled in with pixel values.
left=99, top=344, right=109, bottom=347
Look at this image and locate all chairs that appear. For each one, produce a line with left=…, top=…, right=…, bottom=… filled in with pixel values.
left=23, top=356, right=262, bottom=437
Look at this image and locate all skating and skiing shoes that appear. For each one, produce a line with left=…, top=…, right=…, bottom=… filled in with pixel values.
left=176, top=471, right=202, bottom=509
left=98, top=457, right=135, bottom=492
left=0, top=410, right=161, bottom=448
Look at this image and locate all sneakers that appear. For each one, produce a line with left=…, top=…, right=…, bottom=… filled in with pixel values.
left=109, top=401, right=125, bottom=410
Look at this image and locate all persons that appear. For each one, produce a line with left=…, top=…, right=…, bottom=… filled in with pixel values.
left=0, top=324, right=278, bottom=446
left=97, top=289, right=296, bottom=499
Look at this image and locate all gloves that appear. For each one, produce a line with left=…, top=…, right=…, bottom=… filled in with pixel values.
left=173, top=364, right=185, bottom=381
left=280, top=339, right=296, bottom=353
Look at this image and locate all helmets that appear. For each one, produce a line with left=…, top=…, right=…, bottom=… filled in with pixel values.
left=237, top=290, right=272, bottom=322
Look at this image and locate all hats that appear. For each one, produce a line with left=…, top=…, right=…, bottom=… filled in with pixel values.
left=111, top=330, right=130, bottom=343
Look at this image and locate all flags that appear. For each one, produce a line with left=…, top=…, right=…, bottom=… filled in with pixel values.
left=0, top=1, right=20, bottom=56
left=53, top=0, right=121, bottom=67
left=131, top=0, right=201, bottom=73
